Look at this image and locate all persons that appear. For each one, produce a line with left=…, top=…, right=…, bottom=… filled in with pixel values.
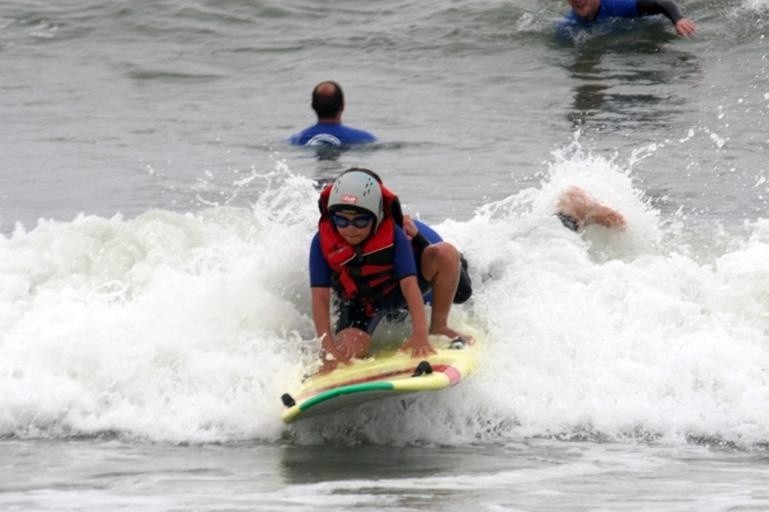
left=553, top=186, right=629, bottom=233
left=309, top=168, right=475, bottom=377
left=287, top=80, right=383, bottom=145
left=550, top=0, right=696, bottom=37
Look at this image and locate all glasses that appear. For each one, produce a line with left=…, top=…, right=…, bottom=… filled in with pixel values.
left=331, top=214, right=373, bottom=228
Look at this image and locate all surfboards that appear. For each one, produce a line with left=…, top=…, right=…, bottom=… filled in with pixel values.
left=281, top=317, right=480, bottom=425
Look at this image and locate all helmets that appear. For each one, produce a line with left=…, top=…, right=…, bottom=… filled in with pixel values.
left=327, top=170, right=384, bottom=236
